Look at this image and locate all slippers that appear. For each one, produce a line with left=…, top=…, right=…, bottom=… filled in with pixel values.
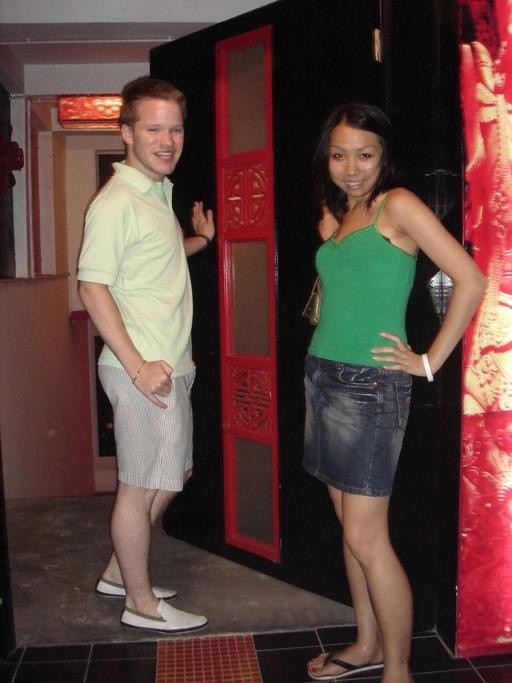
left=308, top=651, right=384, bottom=680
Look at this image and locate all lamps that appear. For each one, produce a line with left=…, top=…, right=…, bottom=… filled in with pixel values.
left=56, top=93, right=123, bottom=129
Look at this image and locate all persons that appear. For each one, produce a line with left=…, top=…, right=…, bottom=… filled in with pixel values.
left=303, top=101, right=486, bottom=683
left=74, top=74, right=216, bottom=635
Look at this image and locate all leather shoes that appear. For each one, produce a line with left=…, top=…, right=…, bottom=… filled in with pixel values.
left=120, top=599, right=208, bottom=635
left=96, top=575, right=177, bottom=601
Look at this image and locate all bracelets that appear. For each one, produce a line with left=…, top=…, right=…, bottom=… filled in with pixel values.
left=192, top=234, right=211, bottom=249
left=131, top=359, right=147, bottom=385
left=421, top=353, right=434, bottom=384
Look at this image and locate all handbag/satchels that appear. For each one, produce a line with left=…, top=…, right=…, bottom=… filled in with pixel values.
left=302, top=293, right=321, bottom=325
